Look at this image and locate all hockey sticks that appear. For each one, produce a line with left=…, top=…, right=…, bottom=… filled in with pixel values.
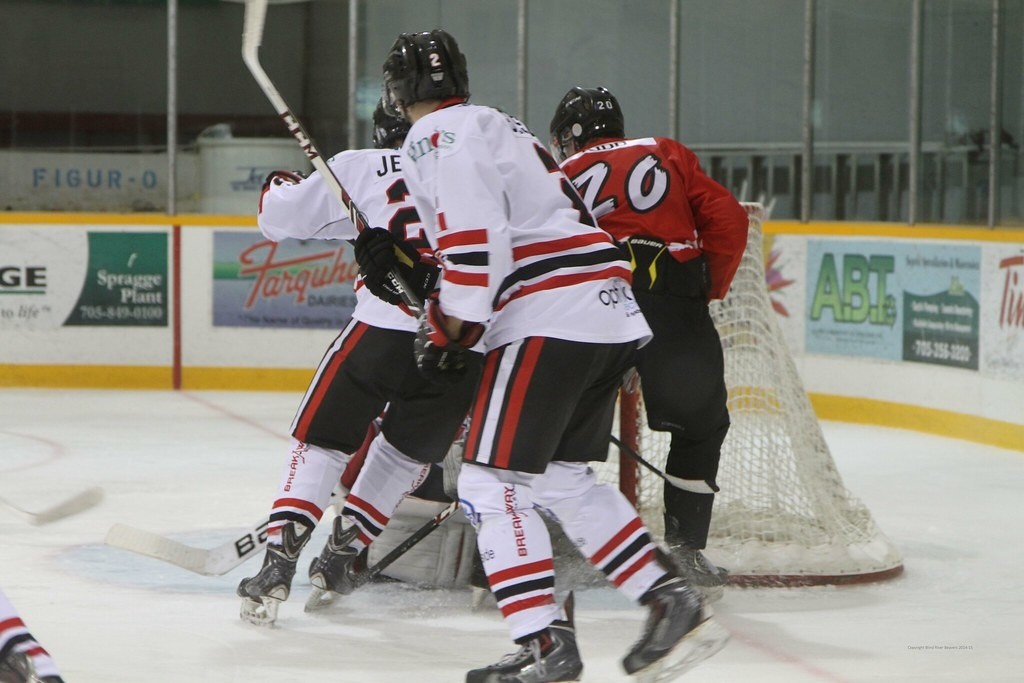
left=368, top=500, right=462, bottom=584
left=609, top=433, right=721, bottom=495
left=102, top=419, right=385, bottom=578
left=0, top=480, right=101, bottom=528
left=239, top=0, right=428, bottom=329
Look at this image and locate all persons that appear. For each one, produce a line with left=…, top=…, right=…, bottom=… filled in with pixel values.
left=381, top=30, right=732, bottom=683
left=238, top=86, right=752, bottom=627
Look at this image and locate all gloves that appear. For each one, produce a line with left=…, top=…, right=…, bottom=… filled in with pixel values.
left=413, top=291, right=487, bottom=390
left=263, top=169, right=306, bottom=185
left=353, top=229, right=439, bottom=309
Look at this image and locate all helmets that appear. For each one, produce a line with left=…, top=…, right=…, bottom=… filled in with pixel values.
left=549, top=86, right=624, bottom=151
left=374, top=90, right=412, bottom=149
left=382, top=30, right=471, bottom=111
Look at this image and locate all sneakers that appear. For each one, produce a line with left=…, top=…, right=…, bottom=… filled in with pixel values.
left=467, top=546, right=494, bottom=610
left=236, top=524, right=314, bottom=629
left=663, top=542, right=731, bottom=603
left=466, top=589, right=583, bottom=683
left=623, top=571, right=732, bottom=683
left=304, top=514, right=368, bottom=612
